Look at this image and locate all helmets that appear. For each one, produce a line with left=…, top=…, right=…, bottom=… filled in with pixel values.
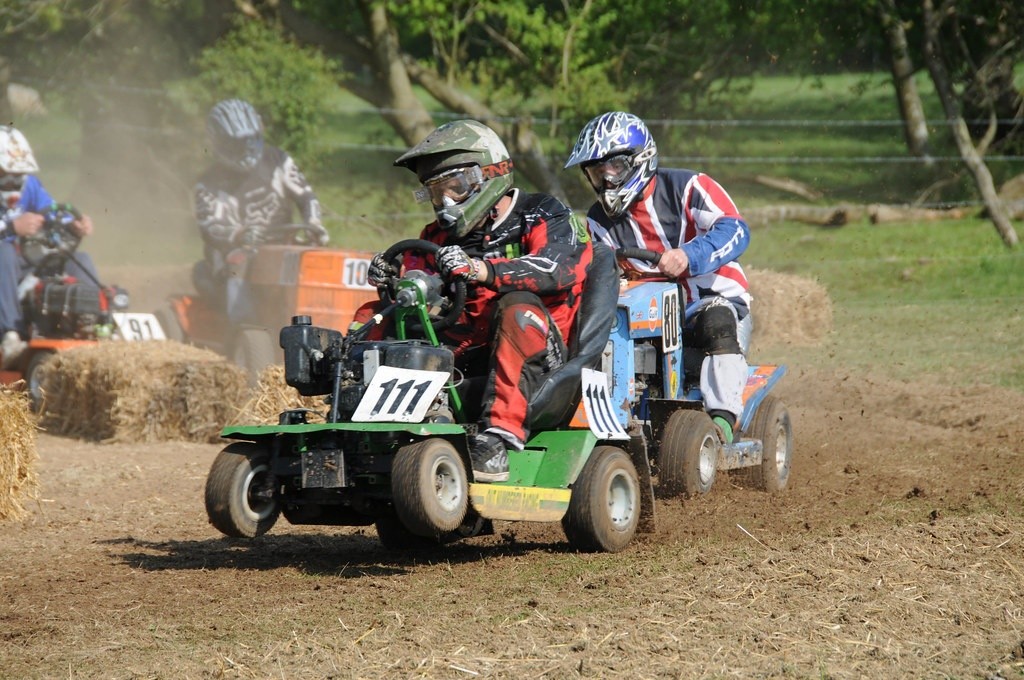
left=207, top=97, right=265, bottom=171
left=563, top=111, right=658, bottom=221
left=0, top=125, right=36, bottom=209
left=393, top=120, right=513, bottom=239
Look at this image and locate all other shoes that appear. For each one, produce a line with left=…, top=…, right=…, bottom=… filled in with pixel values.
left=0, top=331, right=29, bottom=371
left=712, top=423, right=728, bottom=444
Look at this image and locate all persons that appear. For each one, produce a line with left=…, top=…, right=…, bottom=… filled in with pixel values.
left=194, top=101, right=328, bottom=329
left=0, top=124, right=99, bottom=371
left=563, top=112, right=754, bottom=445
left=347, top=120, right=592, bottom=482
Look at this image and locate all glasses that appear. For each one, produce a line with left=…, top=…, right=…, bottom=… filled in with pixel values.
left=424, top=166, right=483, bottom=209
left=585, top=156, right=635, bottom=192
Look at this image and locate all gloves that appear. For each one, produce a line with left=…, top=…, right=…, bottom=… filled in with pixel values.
left=367, top=253, right=401, bottom=287
left=242, top=224, right=267, bottom=243
left=435, top=245, right=480, bottom=283
left=308, top=225, right=330, bottom=246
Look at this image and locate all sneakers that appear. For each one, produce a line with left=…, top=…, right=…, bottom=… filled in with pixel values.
left=472, top=432, right=510, bottom=482
